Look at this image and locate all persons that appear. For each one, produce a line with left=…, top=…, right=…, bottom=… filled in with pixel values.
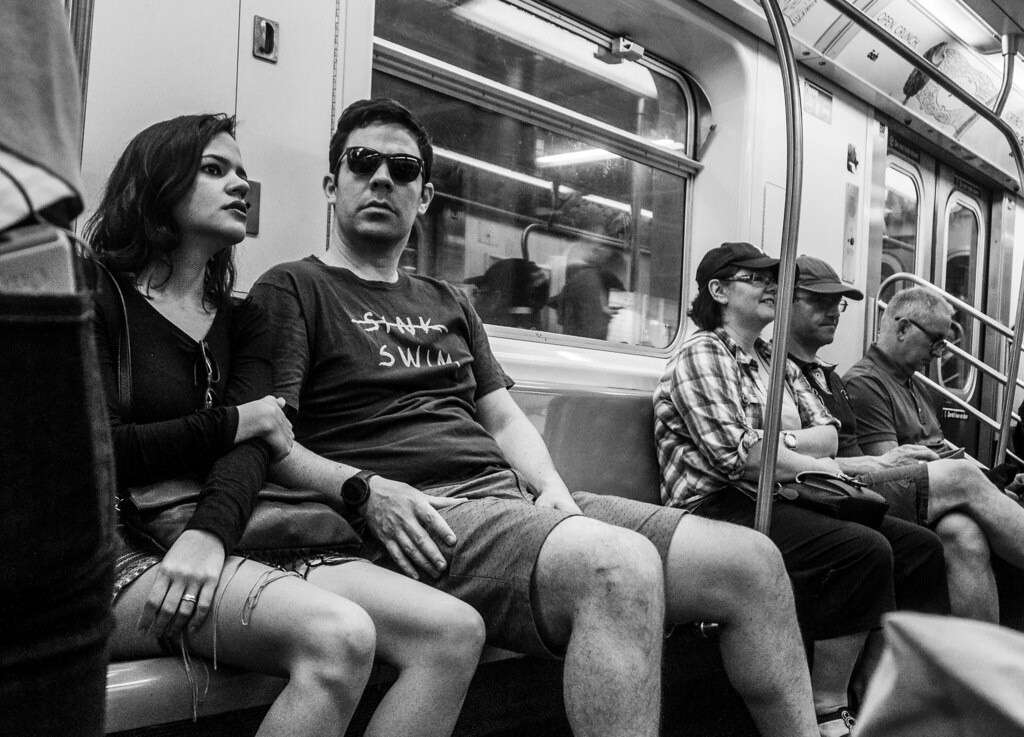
left=840, top=286, right=1024, bottom=503
left=547, top=264, right=626, bottom=340
left=79, top=111, right=487, bottom=737
left=0, top=0, right=117, bottom=736
left=240, top=100, right=823, bottom=736
left=654, top=239, right=955, bottom=727
left=462, top=257, right=549, bottom=330
left=770, top=252, right=1024, bottom=625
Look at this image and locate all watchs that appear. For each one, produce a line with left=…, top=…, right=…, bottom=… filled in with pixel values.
left=781, top=429, right=798, bottom=452
left=340, top=470, right=376, bottom=513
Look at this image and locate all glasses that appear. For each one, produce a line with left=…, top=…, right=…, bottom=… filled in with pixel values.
left=333, top=145, right=426, bottom=190
left=894, top=316, right=947, bottom=358
left=795, top=296, right=848, bottom=313
left=718, top=271, right=777, bottom=287
left=470, top=288, right=494, bottom=299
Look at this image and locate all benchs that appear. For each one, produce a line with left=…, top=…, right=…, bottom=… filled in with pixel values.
left=106, top=386, right=656, bottom=735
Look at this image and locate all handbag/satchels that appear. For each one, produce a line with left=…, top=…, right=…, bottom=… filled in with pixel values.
left=121, top=479, right=365, bottom=554
left=774, top=470, right=891, bottom=530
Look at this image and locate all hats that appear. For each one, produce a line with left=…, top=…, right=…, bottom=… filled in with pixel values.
left=695, top=241, right=801, bottom=291
left=462, top=258, right=549, bottom=309
left=796, top=253, right=864, bottom=301
left=547, top=267, right=628, bottom=310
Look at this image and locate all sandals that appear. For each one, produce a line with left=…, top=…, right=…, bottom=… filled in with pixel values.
left=816, top=707, right=857, bottom=736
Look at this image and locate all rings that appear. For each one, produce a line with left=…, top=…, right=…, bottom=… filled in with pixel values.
left=183, top=594, right=196, bottom=602
left=918, top=459, right=924, bottom=464
